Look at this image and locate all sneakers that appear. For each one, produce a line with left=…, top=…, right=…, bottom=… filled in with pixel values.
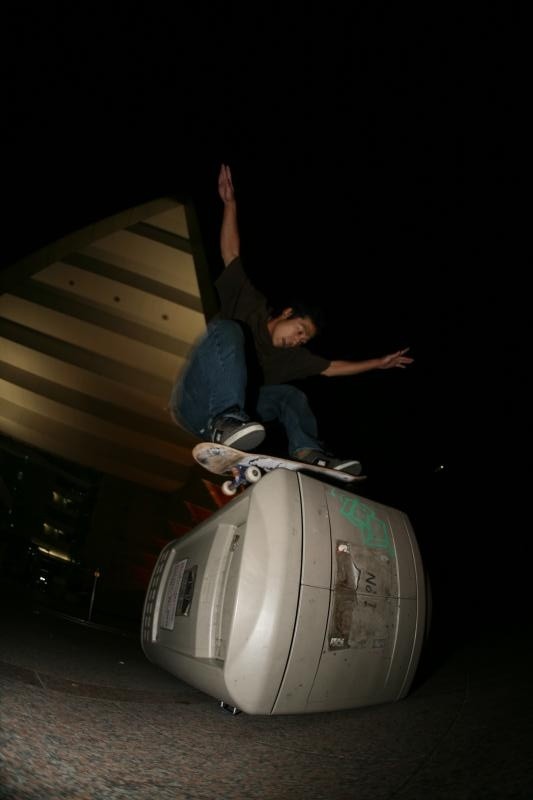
left=215, top=418, right=264, bottom=451
left=305, top=456, right=361, bottom=476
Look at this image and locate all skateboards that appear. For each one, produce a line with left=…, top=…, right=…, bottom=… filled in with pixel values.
left=192, top=442, right=369, bottom=496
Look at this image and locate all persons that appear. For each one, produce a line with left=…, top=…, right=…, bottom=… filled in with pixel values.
left=168, top=163, right=414, bottom=474
